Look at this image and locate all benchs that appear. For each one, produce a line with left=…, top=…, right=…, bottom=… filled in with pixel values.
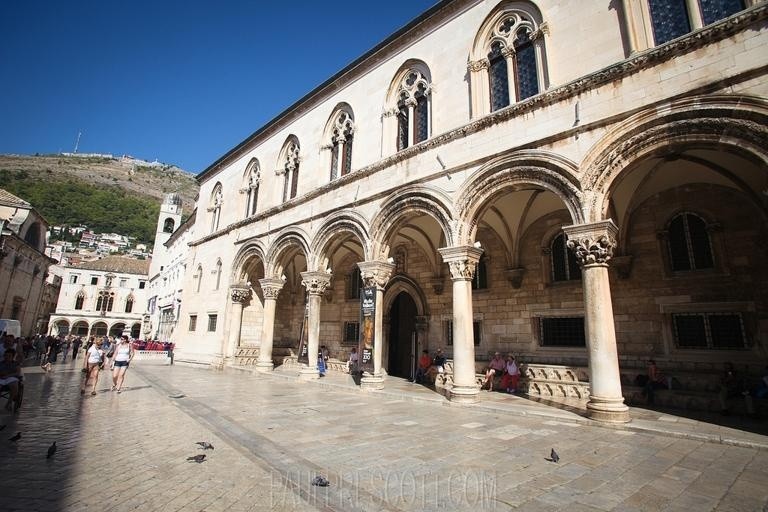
left=433, top=359, right=766, bottom=419
left=234, top=341, right=359, bottom=377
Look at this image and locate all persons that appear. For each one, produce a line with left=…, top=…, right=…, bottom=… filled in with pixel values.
left=0, top=328, right=177, bottom=414
left=483, top=352, right=506, bottom=392
left=717, top=362, right=762, bottom=420
left=349, top=348, right=357, bottom=373
left=423, top=344, right=446, bottom=377
left=501, top=353, right=521, bottom=393
left=638, top=360, right=668, bottom=407
left=412, top=349, right=432, bottom=383
left=320, top=345, right=329, bottom=360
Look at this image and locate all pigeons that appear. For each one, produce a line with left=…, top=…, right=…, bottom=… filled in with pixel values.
left=312, top=475, right=329, bottom=487
left=195, top=441, right=215, bottom=451
left=187, top=454, right=206, bottom=462
left=46, top=441, right=57, bottom=458
left=0, top=425, right=7, bottom=431
left=551, top=448, right=560, bottom=462
left=8, top=432, right=21, bottom=445
